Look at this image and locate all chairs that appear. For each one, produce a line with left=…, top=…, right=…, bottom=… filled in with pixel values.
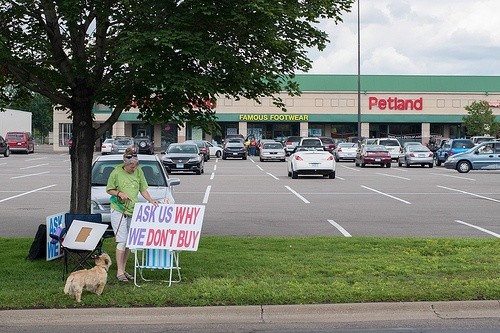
left=142, top=166, right=157, bottom=183
left=129, top=247, right=182, bottom=287
left=103, top=167, right=115, bottom=181
left=49, top=213, right=106, bottom=283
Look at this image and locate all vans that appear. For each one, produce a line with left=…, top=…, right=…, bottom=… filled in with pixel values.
left=5, top=131, right=34, bottom=154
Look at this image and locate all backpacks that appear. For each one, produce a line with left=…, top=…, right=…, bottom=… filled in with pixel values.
left=25, top=224, right=46, bottom=263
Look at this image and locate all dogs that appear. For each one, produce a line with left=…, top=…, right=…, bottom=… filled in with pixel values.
left=63, top=252, right=113, bottom=302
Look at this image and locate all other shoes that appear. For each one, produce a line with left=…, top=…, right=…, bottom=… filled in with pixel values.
left=116, top=272, right=134, bottom=282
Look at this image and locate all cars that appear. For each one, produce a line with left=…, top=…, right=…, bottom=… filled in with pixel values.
left=332, top=143, right=358, bottom=162
left=445, top=141, right=500, bottom=173
left=182, top=140, right=210, bottom=162
left=101, top=139, right=113, bottom=155
left=320, top=137, right=336, bottom=151
left=284, top=145, right=336, bottom=180
left=134, top=135, right=155, bottom=155
left=221, top=134, right=255, bottom=160
left=111, top=137, right=138, bottom=154
left=90, top=154, right=180, bottom=231
left=0, top=135, right=10, bottom=157
left=427, top=135, right=496, bottom=166
left=160, top=143, right=204, bottom=175
left=355, top=138, right=434, bottom=168
left=205, top=141, right=223, bottom=157
left=259, top=142, right=286, bottom=162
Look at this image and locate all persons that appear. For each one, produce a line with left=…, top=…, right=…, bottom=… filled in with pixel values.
left=246, top=138, right=256, bottom=157
left=107, top=148, right=159, bottom=283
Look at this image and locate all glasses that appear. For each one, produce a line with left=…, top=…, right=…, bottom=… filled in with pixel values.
left=124, top=152, right=137, bottom=159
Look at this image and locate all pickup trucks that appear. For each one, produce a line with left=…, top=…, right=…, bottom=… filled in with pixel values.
left=284, top=136, right=302, bottom=152
left=294, top=137, right=325, bottom=153
